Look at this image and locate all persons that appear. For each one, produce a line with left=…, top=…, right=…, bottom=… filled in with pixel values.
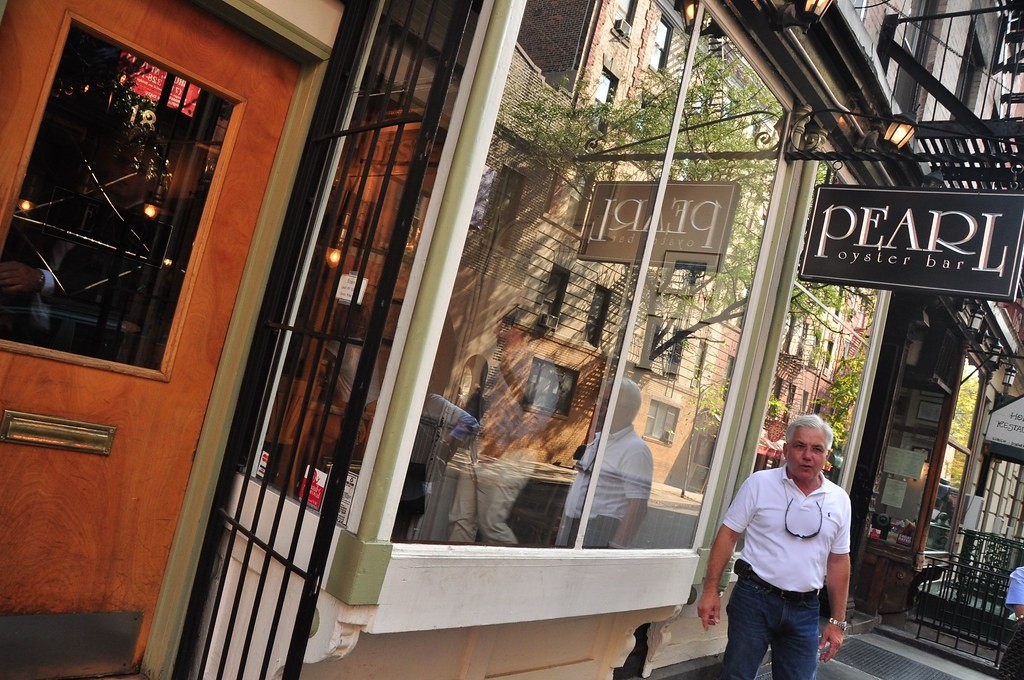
left=996, top=567, right=1024, bottom=680
left=446, top=306, right=560, bottom=544
left=564, top=376, right=654, bottom=549
left=0, top=132, right=164, bottom=358
left=697, top=414, right=852, bottom=680
left=364, top=361, right=480, bottom=519
left=308, top=304, right=380, bottom=467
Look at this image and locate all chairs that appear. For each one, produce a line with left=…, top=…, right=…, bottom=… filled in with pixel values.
left=511, top=483, right=570, bottom=542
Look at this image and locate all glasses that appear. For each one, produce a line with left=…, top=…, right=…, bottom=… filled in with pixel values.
left=785, top=497, right=822, bottom=538
left=576, top=443, right=599, bottom=475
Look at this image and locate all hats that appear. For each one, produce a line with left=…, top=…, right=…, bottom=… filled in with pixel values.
left=493, top=303, right=548, bottom=338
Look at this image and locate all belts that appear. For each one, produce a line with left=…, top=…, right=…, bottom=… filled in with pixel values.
left=751, top=572, right=818, bottom=600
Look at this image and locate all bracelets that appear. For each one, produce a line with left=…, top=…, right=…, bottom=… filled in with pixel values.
left=828, top=617, right=847, bottom=631
left=609, top=540, right=632, bottom=549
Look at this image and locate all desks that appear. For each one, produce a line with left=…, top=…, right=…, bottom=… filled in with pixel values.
left=445, top=452, right=579, bottom=543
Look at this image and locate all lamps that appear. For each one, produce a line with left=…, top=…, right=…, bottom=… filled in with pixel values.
left=999, top=359, right=1017, bottom=386
left=924, top=164, right=944, bottom=188
left=776, top=0, right=833, bottom=34
left=862, top=104, right=921, bottom=156
left=673, top=0, right=724, bottom=38
left=984, top=330, right=1003, bottom=364
left=966, top=302, right=986, bottom=334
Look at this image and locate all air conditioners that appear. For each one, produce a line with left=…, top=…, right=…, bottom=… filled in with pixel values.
left=615, top=20, right=631, bottom=37
left=540, top=314, right=560, bottom=330
left=961, top=494, right=985, bottom=532
left=663, top=431, right=675, bottom=443
